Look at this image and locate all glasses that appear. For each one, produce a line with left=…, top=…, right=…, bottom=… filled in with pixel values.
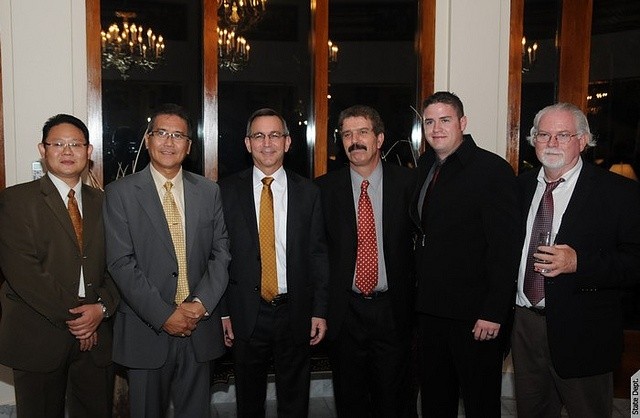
left=534, top=129, right=582, bottom=143
left=42, top=139, right=89, bottom=150
left=246, top=132, right=287, bottom=140
left=149, top=128, right=191, bottom=140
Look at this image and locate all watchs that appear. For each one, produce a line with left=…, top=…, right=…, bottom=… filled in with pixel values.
left=99, top=302, right=111, bottom=319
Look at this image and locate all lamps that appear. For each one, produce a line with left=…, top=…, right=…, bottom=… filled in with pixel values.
left=100, top=2, right=165, bottom=80
left=216, top=26, right=251, bottom=73
left=522, top=36, right=538, bottom=75
left=609, top=162, right=636, bottom=181
left=327, top=41, right=339, bottom=61
left=587, top=76, right=609, bottom=117
left=216, top=0, right=269, bottom=28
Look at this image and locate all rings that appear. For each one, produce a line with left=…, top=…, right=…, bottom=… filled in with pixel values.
left=487, top=332, right=495, bottom=337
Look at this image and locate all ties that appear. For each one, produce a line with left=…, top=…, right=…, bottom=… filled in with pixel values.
left=67, top=188, right=83, bottom=264
left=523, top=176, right=565, bottom=306
left=259, top=176, right=278, bottom=303
left=355, top=179, right=378, bottom=296
left=417, top=163, right=438, bottom=226
left=162, top=181, right=190, bottom=305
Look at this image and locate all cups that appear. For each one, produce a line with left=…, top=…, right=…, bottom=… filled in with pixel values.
left=535, top=230, right=553, bottom=273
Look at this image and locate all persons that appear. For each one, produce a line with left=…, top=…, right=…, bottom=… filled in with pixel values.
left=397, top=91, right=516, bottom=417
left=0, top=114, right=120, bottom=418
left=222, top=108, right=329, bottom=418
left=104, top=111, right=233, bottom=418
left=312, top=105, right=416, bottom=418
left=511, top=101, right=637, bottom=417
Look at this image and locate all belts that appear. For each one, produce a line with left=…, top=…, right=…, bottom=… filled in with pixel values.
left=354, top=290, right=391, bottom=304
left=260, top=295, right=289, bottom=306
left=530, top=307, right=545, bottom=315
